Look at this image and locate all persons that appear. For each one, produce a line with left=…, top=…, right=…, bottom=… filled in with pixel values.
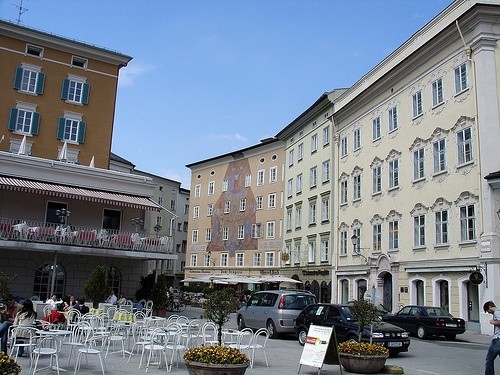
left=12, top=299, right=38, bottom=357
left=41, top=291, right=143, bottom=326
left=0, top=300, right=24, bottom=353
left=482, top=301, right=500, bottom=375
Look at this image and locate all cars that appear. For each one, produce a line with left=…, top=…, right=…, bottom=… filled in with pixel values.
left=384, top=305, right=466, bottom=340
left=295, top=303, right=410, bottom=357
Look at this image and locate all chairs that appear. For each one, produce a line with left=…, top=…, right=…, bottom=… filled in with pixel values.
left=0, top=220, right=170, bottom=252
left=7, top=298, right=270, bottom=374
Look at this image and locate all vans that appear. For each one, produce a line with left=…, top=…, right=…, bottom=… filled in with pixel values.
left=236, top=290, right=316, bottom=338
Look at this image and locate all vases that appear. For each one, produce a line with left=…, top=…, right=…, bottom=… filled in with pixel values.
left=338, top=352, right=388, bottom=373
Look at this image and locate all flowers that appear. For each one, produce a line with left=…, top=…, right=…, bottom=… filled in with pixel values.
left=338, top=340, right=389, bottom=357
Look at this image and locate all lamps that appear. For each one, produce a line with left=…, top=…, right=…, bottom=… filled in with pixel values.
left=351, top=234, right=368, bottom=263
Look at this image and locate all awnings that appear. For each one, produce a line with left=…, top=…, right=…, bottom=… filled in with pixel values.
left=0, top=177, right=163, bottom=213
left=179, top=272, right=304, bottom=285
